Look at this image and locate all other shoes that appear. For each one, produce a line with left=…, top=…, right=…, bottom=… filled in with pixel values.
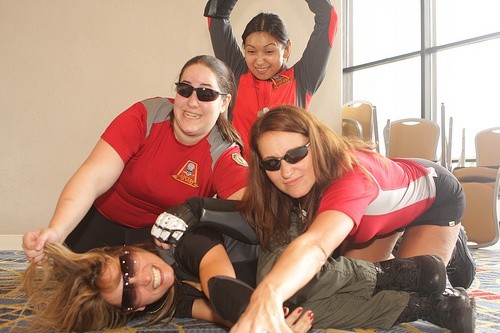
left=445, top=225, right=476, bottom=289
left=392, top=286, right=476, bottom=332
left=372, top=254, right=446, bottom=297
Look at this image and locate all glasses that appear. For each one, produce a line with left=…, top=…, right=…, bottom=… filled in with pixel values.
left=175, top=81, right=228, bottom=102
left=260, top=140, right=312, bottom=172
left=119, top=244, right=140, bottom=313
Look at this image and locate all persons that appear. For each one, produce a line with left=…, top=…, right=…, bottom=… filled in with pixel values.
left=204, top=0, right=338, bottom=166
left=3, top=55, right=478, bottom=333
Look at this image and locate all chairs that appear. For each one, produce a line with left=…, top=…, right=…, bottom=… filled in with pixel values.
left=342, top=100, right=500, bottom=249
